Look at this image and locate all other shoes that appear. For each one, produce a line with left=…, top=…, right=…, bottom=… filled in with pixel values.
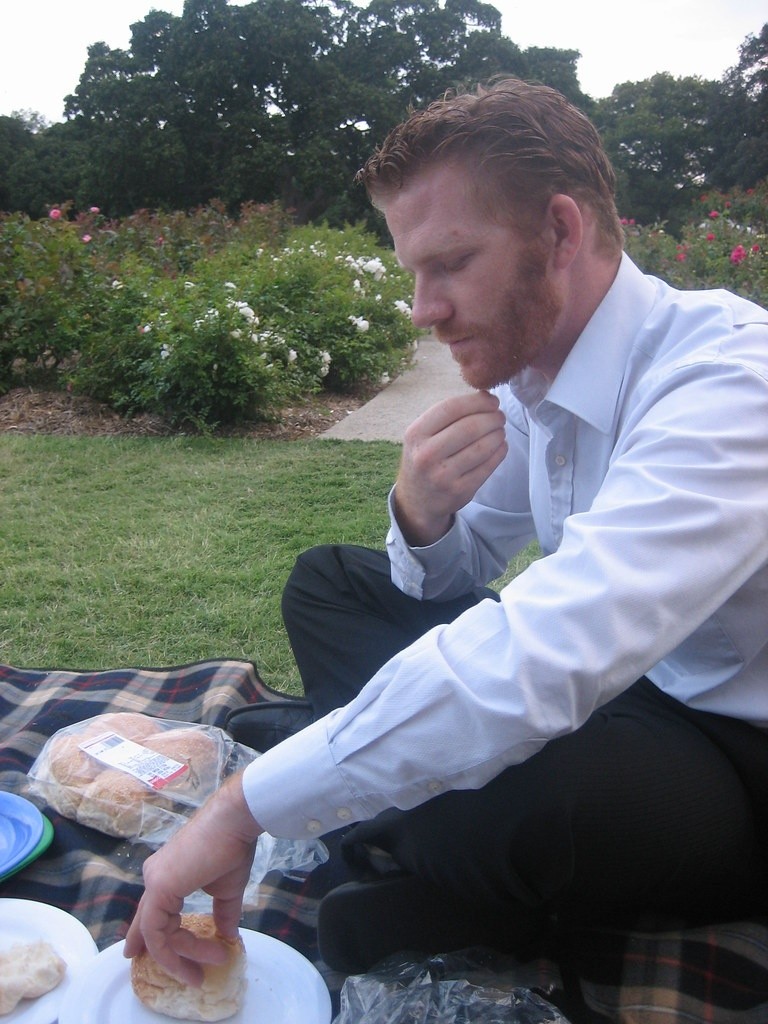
left=219, top=700, right=320, bottom=750
left=317, top=882, right=552, bottom=974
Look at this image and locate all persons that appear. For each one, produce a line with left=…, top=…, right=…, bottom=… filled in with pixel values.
left=124, top=79, right=768, bottom=985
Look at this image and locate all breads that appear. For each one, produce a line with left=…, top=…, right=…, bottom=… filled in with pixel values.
left=50, top=714, right=218, bottom=836
left=131, top=914, right=245, bottom=1022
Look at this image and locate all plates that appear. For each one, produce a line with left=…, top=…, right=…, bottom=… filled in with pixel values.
left=58, top=927, right=332, bottom=1024
left=0, top=898, right=99, bottom=1024
left=0, top=790, right=55, bottom=884
